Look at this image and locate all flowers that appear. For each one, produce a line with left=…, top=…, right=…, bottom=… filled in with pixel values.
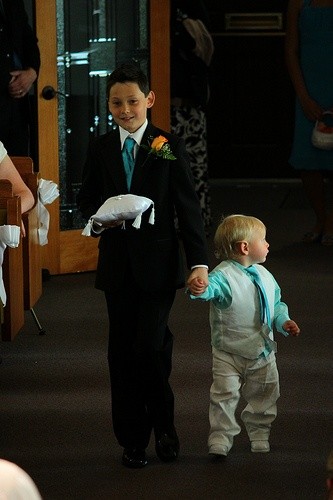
left=150, top=135, right=177, bottom=162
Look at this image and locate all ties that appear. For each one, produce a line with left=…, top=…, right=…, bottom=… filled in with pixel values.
left=121, top=137, right=136, bottom=193
left=244, top=266, right=272, bottom=332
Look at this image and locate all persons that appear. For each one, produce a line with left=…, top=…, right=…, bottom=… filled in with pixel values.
left=284, top=0, right=333, bottom=247
left=78, top=66, right=209, bottom=474
left=0, top=142, right=34, bottom=213
left=0, top=0, right=41, bottom=156
left=185, top=213, right=300, bottom=462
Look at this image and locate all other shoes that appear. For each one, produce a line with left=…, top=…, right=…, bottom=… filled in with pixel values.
left=154, top=426, right=179, bottom=461
left=208, top=443, right=227, bottom=456
left=123, top=446, right=149, bottom=467
left=251, top=440, right=271, bottom=453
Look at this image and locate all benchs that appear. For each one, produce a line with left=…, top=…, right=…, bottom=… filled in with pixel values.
left=11, top=154, right=45, bottom=309
left=0, top=180, right=26, bottom=343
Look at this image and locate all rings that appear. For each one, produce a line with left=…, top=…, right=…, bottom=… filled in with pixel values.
left=19, top=89, right=22, bottom=93
